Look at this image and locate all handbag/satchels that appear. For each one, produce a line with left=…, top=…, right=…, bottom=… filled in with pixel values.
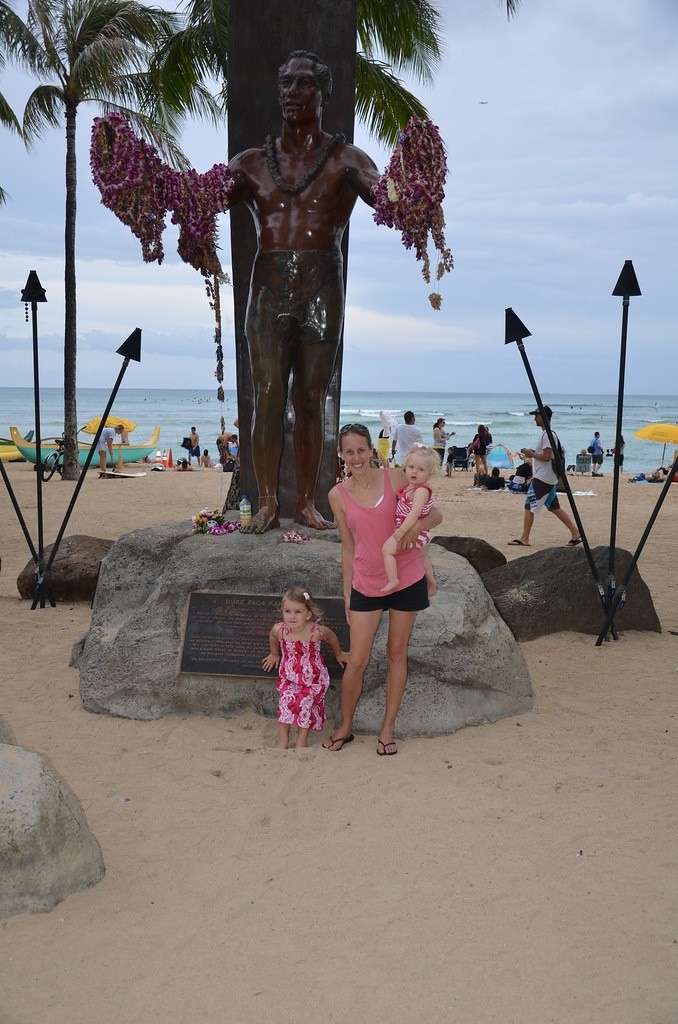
left=588, top=446, right=595, bottom=454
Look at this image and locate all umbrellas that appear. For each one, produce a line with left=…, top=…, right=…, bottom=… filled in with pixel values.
left=81, top=415, right=136, bottom=434
left=635, top=423, right=678, bottom=467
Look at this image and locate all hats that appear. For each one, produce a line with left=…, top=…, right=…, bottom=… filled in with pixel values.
left=529, top=404, right=552, bottom=420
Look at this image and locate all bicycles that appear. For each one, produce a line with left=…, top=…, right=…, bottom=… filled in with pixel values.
left=41, top=424, right=87, bottom=483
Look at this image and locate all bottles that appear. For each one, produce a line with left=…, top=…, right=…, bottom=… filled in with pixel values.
left=239, top=495, right=252, bottom=527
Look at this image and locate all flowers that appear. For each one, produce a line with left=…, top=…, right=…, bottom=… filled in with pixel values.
left=191, top=506, right=225, bottom=533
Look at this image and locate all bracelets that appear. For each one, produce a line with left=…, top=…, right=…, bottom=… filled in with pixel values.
left=393, top=450, right=395, bottom=452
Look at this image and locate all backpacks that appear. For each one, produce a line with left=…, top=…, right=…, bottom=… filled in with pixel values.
left=542, top=431, right=564, bottom=477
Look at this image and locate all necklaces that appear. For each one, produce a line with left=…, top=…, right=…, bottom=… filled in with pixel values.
left=351, top=476, right=374, bottom=494
left=265, top=133, right=345, bottom=193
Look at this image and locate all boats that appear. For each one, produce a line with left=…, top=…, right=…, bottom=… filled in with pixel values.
left=10, top=425, right=161, bottom=473
left=0, top=429, right=35, bottom=462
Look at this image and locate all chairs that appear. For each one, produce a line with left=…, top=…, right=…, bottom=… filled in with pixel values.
left=451, top=447, right=469, bottom=471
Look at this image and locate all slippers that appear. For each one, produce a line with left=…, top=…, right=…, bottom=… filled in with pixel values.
left=376, top=735, right=397, bottom=755
left=508, top=538, right=530, bottom=546
left=565, top=538, right=582, bottom=549
left=321, top=731, right=354, bottom=751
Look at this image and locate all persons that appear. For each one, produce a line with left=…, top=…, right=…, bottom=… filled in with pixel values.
left=200, top=449, right=212, bottom=467
left=469, top=425, right=535, bottom=494
left=96, top=49, right=447, bottom=534
left=97, top=425, right=125, bottom=477
left=612, top=434, right=625, bottom=473
left=378, top=408, right=398, bottom=468
left=645, top=467, right=671, bottom=481
left=337, top=444, right=380, bottom=482
left=590, top=432, right=604, bottom=477
left=380, top=446, right=437, bottom=597
left=433, top=418, right=457, bottom=477
left=216, top=419, right=239, bottom=469
left=188, top=427, right=200, bottom=466
left=176, top=458, right=194, bottom=471
left=392, top=411, right=422, bottom=472
left=261, top=587, right=349, bottom=751
left=508, top=405, right=583, bottom=547
left=322, top=424, right=443, bottom=753
left=606, top=449, right=613, bottom=456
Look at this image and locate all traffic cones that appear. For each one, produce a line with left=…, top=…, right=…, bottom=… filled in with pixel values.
left=168, top=448, right=174, bottom=469
left=155, top=448, right=162, bottom=465
left=161, top=449, right=169, bottom=467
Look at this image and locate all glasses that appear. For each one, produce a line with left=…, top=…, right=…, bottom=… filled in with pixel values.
left=440, top=422, right=445, bottom=424
left=340, top=423, right=368, bottom=435
